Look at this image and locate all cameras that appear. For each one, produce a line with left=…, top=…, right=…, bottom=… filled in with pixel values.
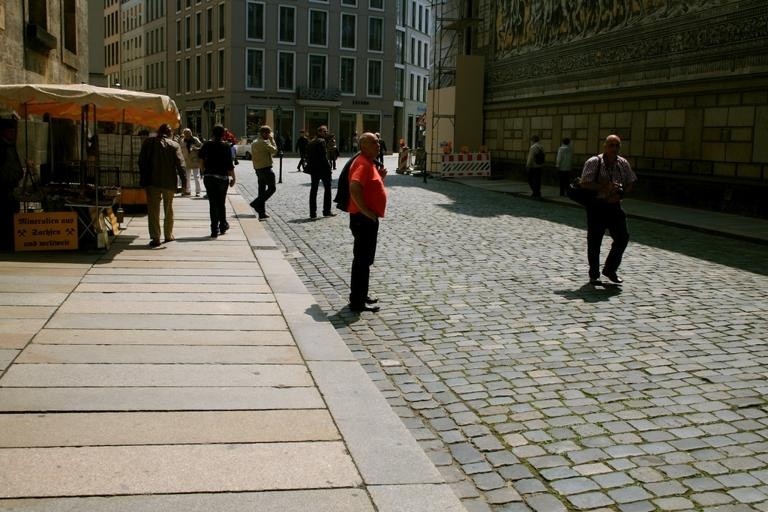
left=614, top=186, right=624, bottom=196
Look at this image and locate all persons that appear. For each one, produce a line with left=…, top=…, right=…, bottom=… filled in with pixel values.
left=348, top=132, right=386, bottom=311
left=0, top=118, right=24, bottom=253
left=180, top=123, right=385, bottom=238
left=581, top=135, right=639, bottom=284
left=555, top=138, right=573, bottom=197
left=138, top=124, right=187, bottom=247
left=526, top=135, right=544, bottom=196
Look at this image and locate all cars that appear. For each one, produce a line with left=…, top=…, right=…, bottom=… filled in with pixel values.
left=231, top=133, right=281, bottom=160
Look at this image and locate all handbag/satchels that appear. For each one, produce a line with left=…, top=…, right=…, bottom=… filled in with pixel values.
left=568, top=177, right=597, bottom=205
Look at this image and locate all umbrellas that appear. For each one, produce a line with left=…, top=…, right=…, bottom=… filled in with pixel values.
left=0, top=81, right=182, bottom=190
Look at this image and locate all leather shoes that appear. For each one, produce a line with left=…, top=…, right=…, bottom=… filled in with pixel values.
left=351, top=297, right=380, bottom=311
left=602, top=267, right=623, bottom=283
left=590, top=277, right=601, bottom=284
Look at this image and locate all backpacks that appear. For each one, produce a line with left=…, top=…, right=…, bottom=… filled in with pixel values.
left=536, top=146, right=544, bottom=164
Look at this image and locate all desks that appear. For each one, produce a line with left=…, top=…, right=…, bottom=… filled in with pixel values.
left=65, top=196, right=116, bottom=246
